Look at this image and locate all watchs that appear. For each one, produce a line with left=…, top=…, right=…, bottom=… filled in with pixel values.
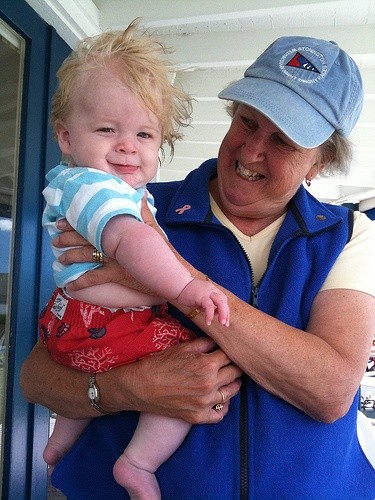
left=87, top=373, right=117, bottom=417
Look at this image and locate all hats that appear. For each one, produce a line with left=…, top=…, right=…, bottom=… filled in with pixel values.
left=218, top=36, right=364, bottom=149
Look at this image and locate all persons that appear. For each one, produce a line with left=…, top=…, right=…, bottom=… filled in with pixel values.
left=38, top=17, right=230, bottom=500
left=21, top=36, right=375, bottom=500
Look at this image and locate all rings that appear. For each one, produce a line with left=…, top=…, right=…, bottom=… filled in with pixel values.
left=216, top=389, right=225, bottom=410
left=93, top=247, right=103, bottom=264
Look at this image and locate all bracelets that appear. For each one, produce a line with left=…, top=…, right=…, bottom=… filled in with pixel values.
left=185, top=275, right=212, bottom=318
